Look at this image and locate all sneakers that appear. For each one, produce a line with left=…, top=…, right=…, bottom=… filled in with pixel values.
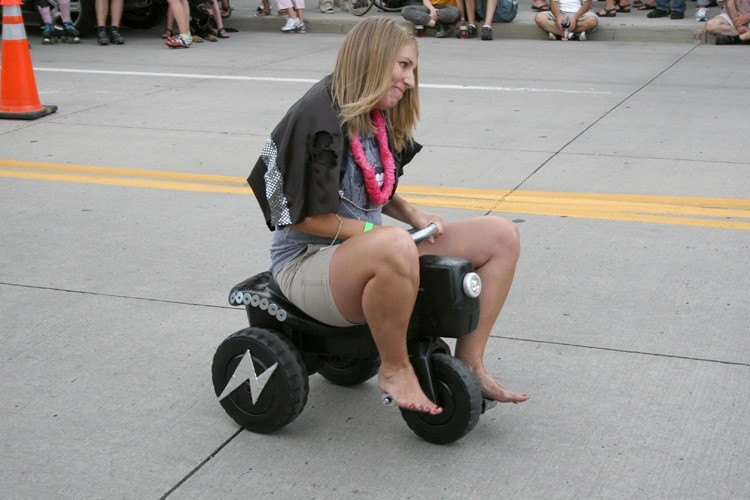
left=295, top=23, right=306, bottom=34
left=282, top=17, right=303, bottom=31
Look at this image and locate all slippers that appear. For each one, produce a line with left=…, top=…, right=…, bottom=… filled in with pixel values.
left=633, top=2, right=643, bottom=7
left=531, top=5, right=551, bottom=11
left=638, top=3, right=656, bottom=9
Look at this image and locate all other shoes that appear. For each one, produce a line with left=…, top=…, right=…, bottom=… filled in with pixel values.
left=715, top=35, right=739, bottom=45
left=468, top=24, right=478, bottom=37
left=435, top=21, right=452, bottom=37
left=108, top=26, right=125, bottom=44
left=481, top=26, right=493, bottom=39
left=162, top=28, right=173, bottom=38
left=324, top=8, right=334, bottom=13
left=670, top=10, right=684, bottom=19
left=570, top=30, right=586, bottom=41
left=60, top=22, right=81, bottom=44
left=96, top=26, right=110, bottom=44
left=42, top=23, right=58, bottom=44
left=548, top=32, right=561, bottom=40
left=256, top=10, right=273, bottom=17
left=695, top=6, right=709, bottom=22
left=165, top=35, right=192, bottom=48
left=647, top=9, right=670, bottom=18
left=217, top=28, right=229, bottom=38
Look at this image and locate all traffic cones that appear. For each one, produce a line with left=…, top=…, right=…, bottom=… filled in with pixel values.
left=0, top=0, right=58, bottom=121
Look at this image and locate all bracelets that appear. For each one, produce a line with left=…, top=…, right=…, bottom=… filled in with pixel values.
left=363, top=221, right=374, bottom=232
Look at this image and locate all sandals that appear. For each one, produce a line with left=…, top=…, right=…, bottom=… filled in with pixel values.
left=614, top=2, right=631, bottom=12
left=596, top=7, right=617, bottom=17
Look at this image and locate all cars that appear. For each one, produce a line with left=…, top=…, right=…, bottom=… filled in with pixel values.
left=0, top=0, right=172, bottom=38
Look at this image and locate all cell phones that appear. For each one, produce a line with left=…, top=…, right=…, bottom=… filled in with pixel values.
left=561, top=23, right=570, bottom=28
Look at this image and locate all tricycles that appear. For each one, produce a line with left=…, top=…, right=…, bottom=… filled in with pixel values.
left=208, top=222, right=499, bottom=445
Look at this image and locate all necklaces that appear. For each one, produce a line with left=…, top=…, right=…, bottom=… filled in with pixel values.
left=352, top=109, right=396, bottom=208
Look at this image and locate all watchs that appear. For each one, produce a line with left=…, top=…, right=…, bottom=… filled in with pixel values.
left=461, top=17, right=465, bottom=20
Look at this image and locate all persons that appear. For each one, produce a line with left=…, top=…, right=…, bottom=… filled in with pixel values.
left=523, top=0, right=750, bottom=46
left=31, top=0, right=234, bottom=48
left=400, top=0, right=498, bottom=40
left=251, top=0, right=364, bottom=35
left=247, top=13, right=530, bottom=415
